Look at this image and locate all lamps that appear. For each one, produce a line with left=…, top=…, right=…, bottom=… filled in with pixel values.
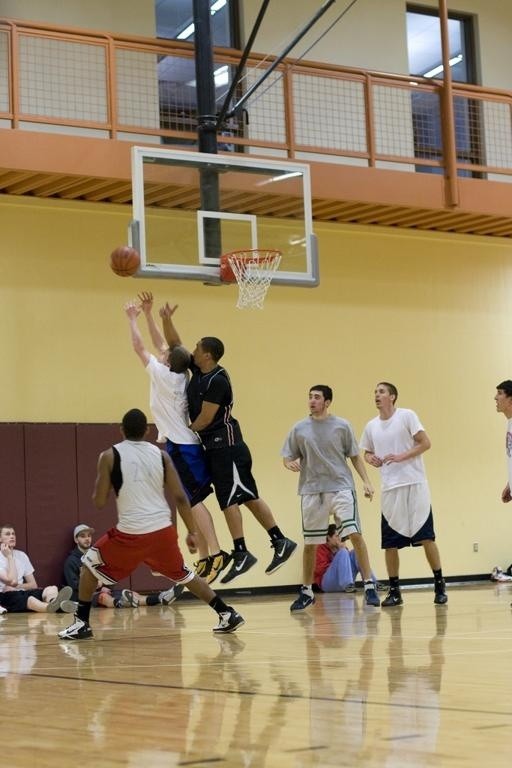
left=173, top=0, right=229, bottom=41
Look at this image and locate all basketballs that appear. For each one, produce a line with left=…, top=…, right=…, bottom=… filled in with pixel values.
left=108, top=247, right=138, bottom=275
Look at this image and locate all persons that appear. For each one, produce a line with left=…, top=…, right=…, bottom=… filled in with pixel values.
left=278, top=384, right=381, bottom=615
left=313, top=523, right=390, bottom=593
left=158, top=300, right=299, bottom=586
left=64, top=524, right=184, bottom=609
left=55, top=408, right=248, bottom=643
left=0, top=522, right=79, bottom=615
left=493, top=379, right=512, bottom=606
left=121, top=289, right=234, bottom=586
left=359, top=381, right=447, bottom=607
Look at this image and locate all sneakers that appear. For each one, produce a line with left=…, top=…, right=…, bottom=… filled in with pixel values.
left=290, top=586, right=316, bottom=614
left=122, top=589, right=139, bottom=608
left=364, top=581, right=402, bottom=608
left=344, top=583, right=356, bottom=593
left=212, top=607, right=244, bottom=633
left=265, top=538, right=298, bottom=575
left=58, top=615, right=95, bottom=640
left=47, top=586, right=79, bottom=614
left=434, top=579, right=447, bottom=604
left=193, top=550, right=257, bottom=585
left=162, top=584, right=184, bottom=605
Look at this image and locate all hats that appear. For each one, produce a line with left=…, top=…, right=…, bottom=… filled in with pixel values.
left=73, top=524, right=94, bottom=537
